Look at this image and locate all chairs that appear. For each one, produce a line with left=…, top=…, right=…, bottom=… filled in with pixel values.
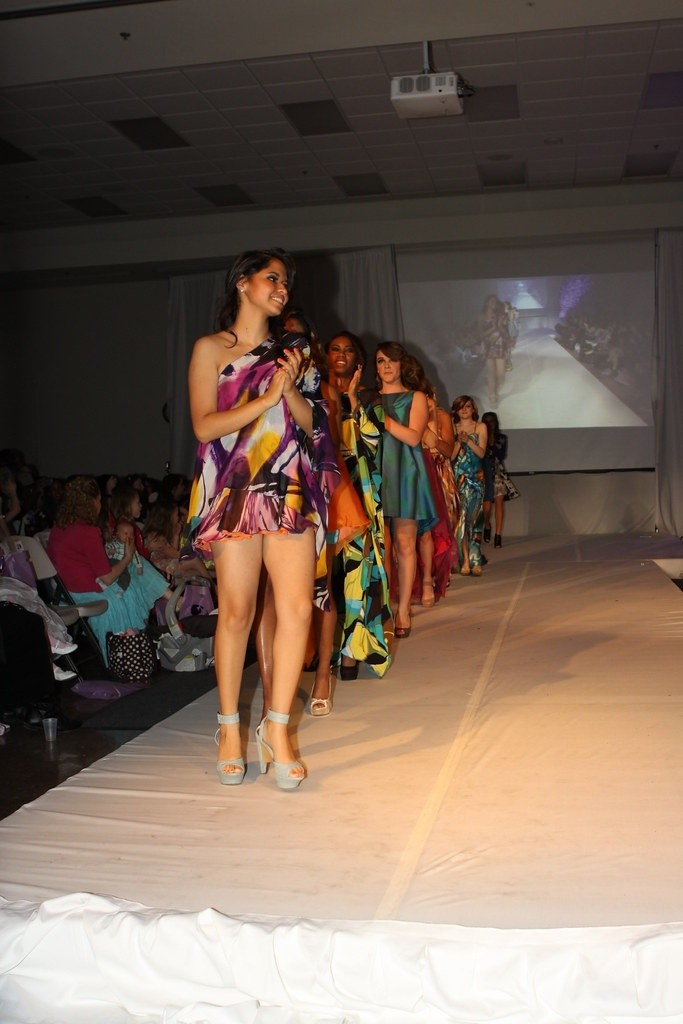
left=3, top=535, right=105, bottom=684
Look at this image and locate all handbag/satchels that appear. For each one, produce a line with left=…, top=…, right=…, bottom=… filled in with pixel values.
left=0, top=515, right=36, bottom=588
left=71, top=681, right=149, bottom=698
left=105, top=631, right=155, bottom=681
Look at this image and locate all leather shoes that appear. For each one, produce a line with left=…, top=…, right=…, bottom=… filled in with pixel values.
left=26, top=707, right=81, bottom=730
left=1, top=705, right=28, bottom=724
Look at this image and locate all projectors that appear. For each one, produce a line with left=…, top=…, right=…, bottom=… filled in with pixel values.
left=390, top=71, right=464, bottom=119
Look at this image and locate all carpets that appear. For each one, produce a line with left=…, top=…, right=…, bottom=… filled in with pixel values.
left=81, top=649, right=258, bottom=731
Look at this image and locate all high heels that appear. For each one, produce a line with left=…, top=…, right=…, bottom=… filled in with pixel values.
left=341, top=651, right=359, bottom=680
left=309, top=675, right=333, bottom=716
left=303, top=651, right=319, bottom=671
left=461, top=558, right=470, bottom=576
left=421, top=579, right=435, bottom=608
left=484, top=524, right=491, bottom=543
left=395, top=609, right=411, bottom=638
left=494, top=533, right=502, bottom=548
left=472, top=564, right=482, bottom=576
left=214, top=711, right=245, bottom=784
left=254, top=709, right=305, bottom=789
left=384, top=631, right=394, bottom=657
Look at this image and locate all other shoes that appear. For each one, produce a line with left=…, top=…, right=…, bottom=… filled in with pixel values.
left=0, top=722, right=10, bottom=735
left=175, top=597, right=185, bottom=612
left=51, top=640, right=78, bottom=654
left=54, top=667, right=77, bottom=681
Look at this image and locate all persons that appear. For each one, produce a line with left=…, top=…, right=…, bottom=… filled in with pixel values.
left=253, top=312, right=519, bottom=722
left=429, top=294, right=520, bottom=408
left=555, top=314, right=653, bottom=390
left=182, top=245, right=343, bottom=789
left=1, top=467, right=221, bottom=740
